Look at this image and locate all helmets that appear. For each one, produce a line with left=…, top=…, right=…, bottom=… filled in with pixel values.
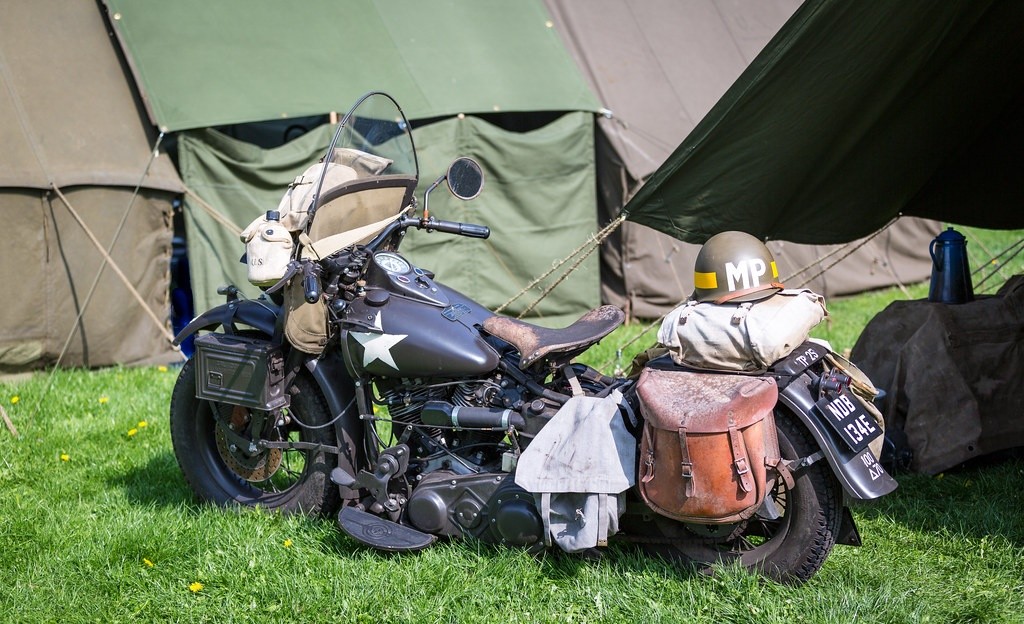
left=694, top=230, right=785, bottom=304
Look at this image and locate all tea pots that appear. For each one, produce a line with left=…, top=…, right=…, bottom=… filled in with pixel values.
left=930, top=227, right=974, bottom=304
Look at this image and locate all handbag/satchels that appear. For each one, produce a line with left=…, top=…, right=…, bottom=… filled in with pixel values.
left=635, top=367, right=795, bottom=525
left=514, top=365, right=635, bottom=553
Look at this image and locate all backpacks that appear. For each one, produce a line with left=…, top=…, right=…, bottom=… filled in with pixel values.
left=656, top=288, right=831, bottom=375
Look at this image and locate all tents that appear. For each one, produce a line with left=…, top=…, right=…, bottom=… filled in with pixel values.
left=620, top=0, right=1024, bottom=247
left=0, top=0, right=947, bottom=369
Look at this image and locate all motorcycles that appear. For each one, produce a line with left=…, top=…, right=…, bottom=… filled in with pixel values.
left=170, top=90, right=899, bottom=589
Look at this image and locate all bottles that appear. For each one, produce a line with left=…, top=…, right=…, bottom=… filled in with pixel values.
left=246, top=210, right=293, bottom=286
